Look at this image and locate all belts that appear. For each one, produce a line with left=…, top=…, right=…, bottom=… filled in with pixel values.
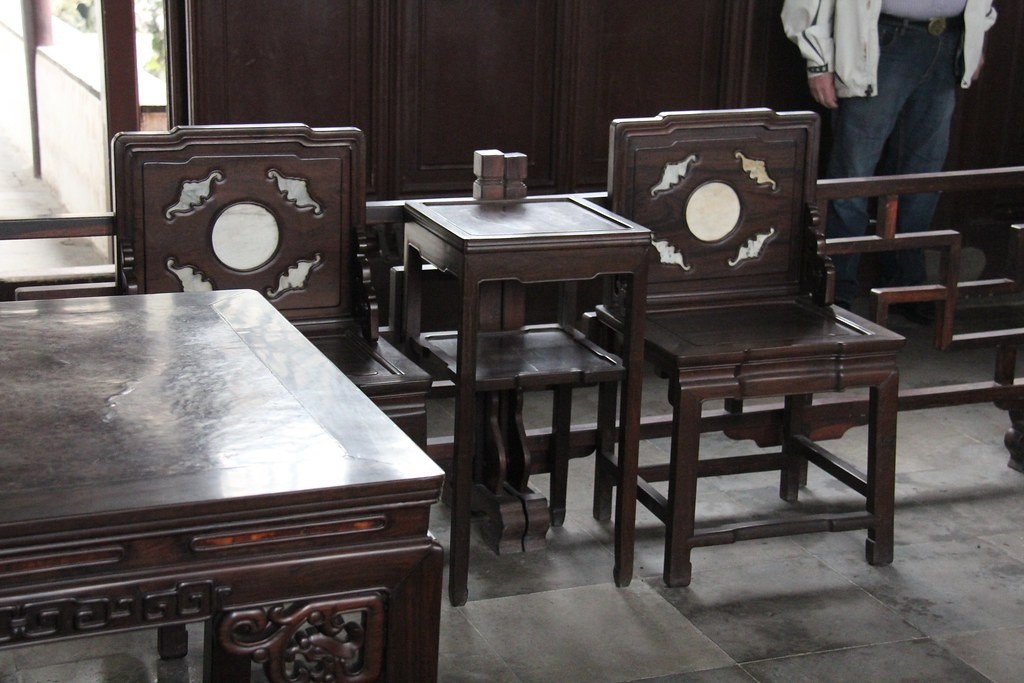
left=879, top=13, right=964, bottom=35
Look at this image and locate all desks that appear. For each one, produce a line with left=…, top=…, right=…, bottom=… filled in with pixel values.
left=0, top=289, right=447, bottom=683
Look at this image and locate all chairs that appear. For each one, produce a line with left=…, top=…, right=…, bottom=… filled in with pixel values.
left=593, top=107, right=908, bottom=589
left=110, top=122, right=430, bottom=660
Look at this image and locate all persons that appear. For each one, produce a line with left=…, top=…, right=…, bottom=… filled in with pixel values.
left=781, top=0, right=1000, bottom=320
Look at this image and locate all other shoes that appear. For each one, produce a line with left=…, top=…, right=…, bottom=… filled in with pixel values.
left=890, top=301, right=938, bottom=326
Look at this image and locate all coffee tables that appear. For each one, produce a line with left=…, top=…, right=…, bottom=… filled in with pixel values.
left=400, top=193, right=653, bottom=607
